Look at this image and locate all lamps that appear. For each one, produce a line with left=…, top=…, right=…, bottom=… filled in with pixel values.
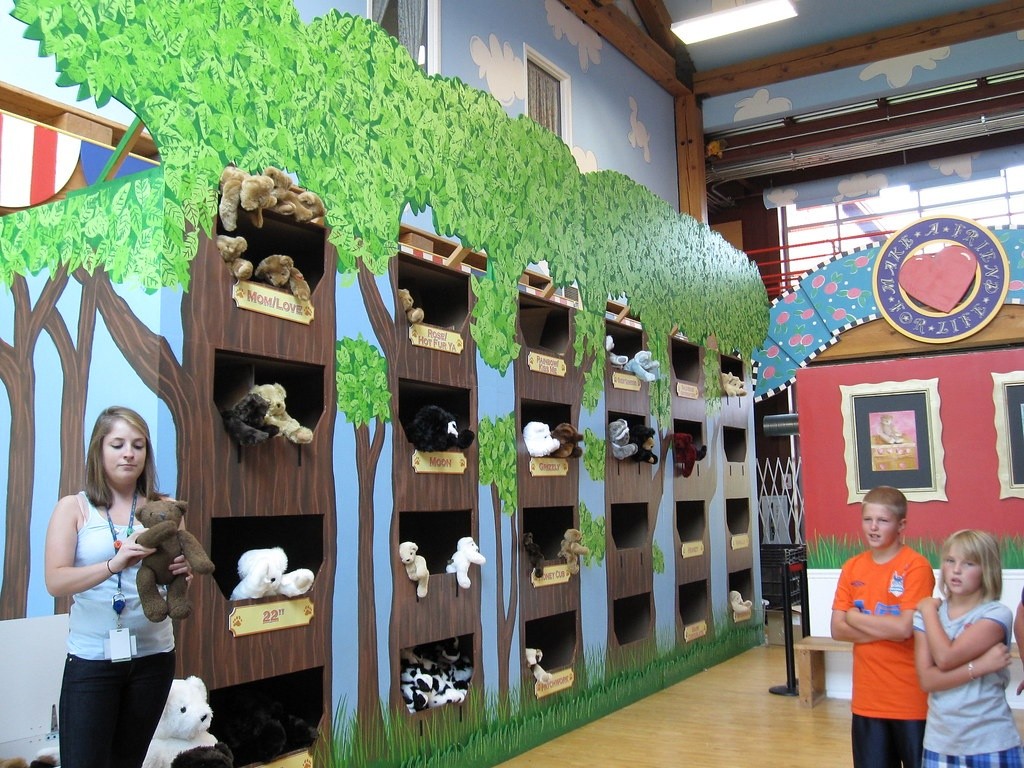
left=670, top=0, right=799, bottom=45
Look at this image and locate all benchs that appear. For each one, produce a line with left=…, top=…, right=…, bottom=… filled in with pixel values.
left=793, top=635, right=1021, bottom=710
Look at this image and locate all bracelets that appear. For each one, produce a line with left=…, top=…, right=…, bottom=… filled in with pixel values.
left=968, top=660, right=976, bottom=680
left=107, top=559, right=120, bottom=575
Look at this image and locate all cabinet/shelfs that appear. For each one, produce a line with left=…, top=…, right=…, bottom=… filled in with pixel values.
left=182, top=155, right=756, bottom=768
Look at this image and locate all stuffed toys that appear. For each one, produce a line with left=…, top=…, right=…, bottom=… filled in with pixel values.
left=0, top=747, right=61, bottom=768
left=401, top=637, right=473, bottom=714
left=408, top=405, right=475, bottom=451
left=523, top=422, right=584, bottom=457
left=674, top=433, right=708, bottom=478
left=217, top=165, right=324, bottom=301
left=609, top=419, right=658, bottom=464
left=399, top=542, right=429, bottom=597
left=446, top=537, right=485, bottom=588
left=558, top=528, right=588, bottom=575
left=142, top=675, right=319, bottom=768
left=229, top=547, right=314, bottom=601
left=223, top=383, right=314, bottom=446
left=134, top=500, right=214, bottom=623
left=399, top=288, right=425, bottom=324
left=722, top=371, right=748, bottom=397
left=731, top=590, right=753, bottom=614
left=524, top=533, right=544, bottom=578
left=606, top=336, right=660, bottom=382
left=526, top=648, right=552, bottom=684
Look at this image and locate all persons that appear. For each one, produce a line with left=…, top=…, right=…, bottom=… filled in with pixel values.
left=45, top=407, right=192, bottom=768
left=830, top=487, right=936, bottom=768
left=912, top=530, right=1024, bottom=768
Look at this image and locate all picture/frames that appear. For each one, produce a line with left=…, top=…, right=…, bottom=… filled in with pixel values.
left=990, top=370, right=1024, bottom=500
left=837, top=377, right=948, bottom=505
